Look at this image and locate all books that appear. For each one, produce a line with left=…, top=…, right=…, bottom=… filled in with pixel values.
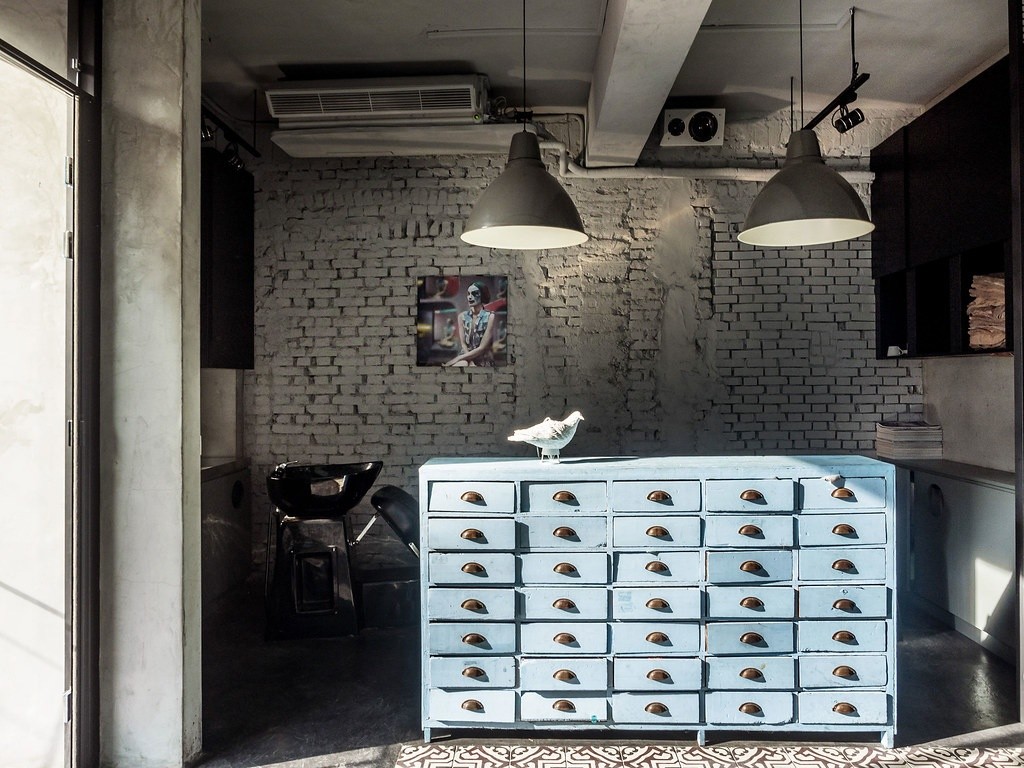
left=875, top=419, right=943, bottom=460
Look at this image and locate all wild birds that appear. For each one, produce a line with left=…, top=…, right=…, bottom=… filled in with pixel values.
left=507, top=410, right=586, bottom=450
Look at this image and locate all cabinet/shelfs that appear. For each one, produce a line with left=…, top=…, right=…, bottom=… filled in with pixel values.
left=870, top=50, right=1014, bottom=360
left=419, top=455, right=898, bottom=748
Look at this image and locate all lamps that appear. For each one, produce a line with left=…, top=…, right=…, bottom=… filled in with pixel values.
left=831, top=104, right=865, bottom=134
left=460, top=0, right=587, bottom=250
left=737, top=0, right=875, bottom=246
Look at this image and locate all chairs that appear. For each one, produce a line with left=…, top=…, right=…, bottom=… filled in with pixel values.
left=261, top=460, right=384, bottom=643
left=370, top=485, right=419, bottom=561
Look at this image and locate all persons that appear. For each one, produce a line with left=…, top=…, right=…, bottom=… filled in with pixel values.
left=439, top=279, right=507, bottom=371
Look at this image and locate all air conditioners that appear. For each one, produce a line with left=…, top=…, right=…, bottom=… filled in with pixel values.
left=266, top=75, right=491, bottom=127
